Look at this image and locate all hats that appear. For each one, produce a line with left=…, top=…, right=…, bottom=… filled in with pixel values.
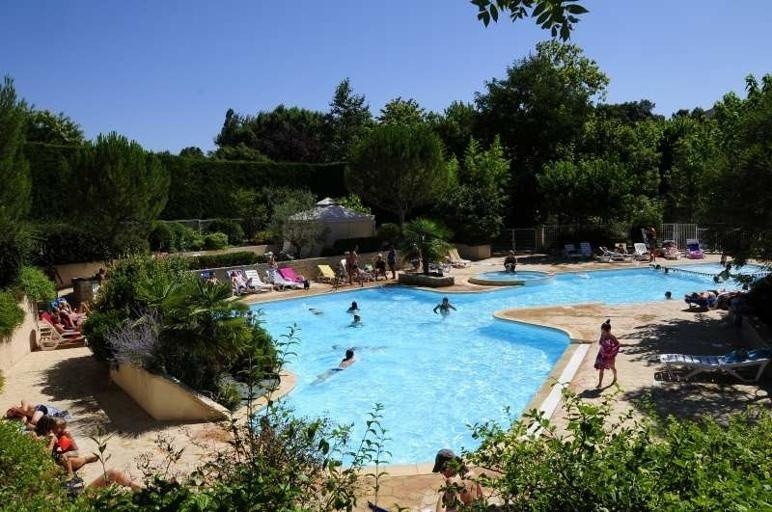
left=433, top=448, right=455, bottom=473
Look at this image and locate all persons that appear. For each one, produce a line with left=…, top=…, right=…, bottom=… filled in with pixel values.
left=433, top=297, right=456, bottom=316
left=51, top=301, right=93, bottom=338
left=205, top=243, right=421, bottom=295
left=435, top=456, right=483, bottom=512
left=594, top=319, right=620, bottom=388
left=2, top=400, right=144, bottom=498
left=504, top=250, right=517, bottom=273
left=616, top=225, right=772, bottom=320
left=310, top=347, right=356, bottom=386
left=346, top=301, right=362, bottom=327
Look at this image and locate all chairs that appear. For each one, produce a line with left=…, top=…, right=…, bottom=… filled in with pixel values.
left=200, top=267, right=308, bottom=294
left=317, top=264, right=336, bottom=284
left=35, top=312, right=85, bottom=351
left=686, top=295, right=716, bottom=311
left=659, top=347, right=772, bottom=384
left=564, top=238, right=704, bottom=262
